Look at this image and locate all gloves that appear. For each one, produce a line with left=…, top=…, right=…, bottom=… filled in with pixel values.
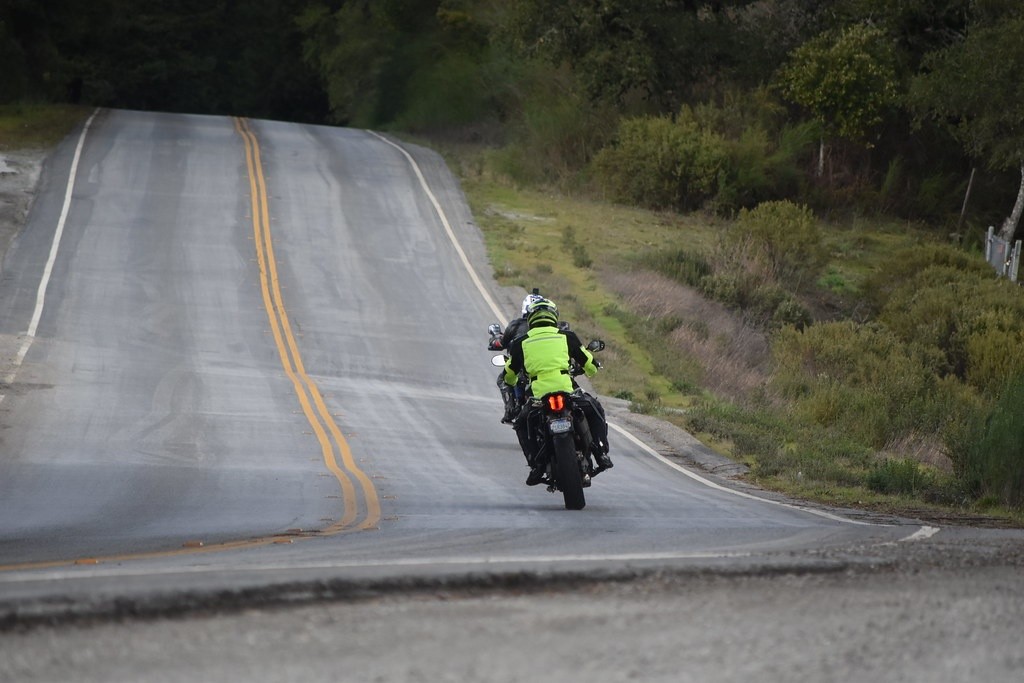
left=489, top=336, right=501, bottom=349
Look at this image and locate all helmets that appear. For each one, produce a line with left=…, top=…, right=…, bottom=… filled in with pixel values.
left=492, top=325, right=500, bottom=334
left=522, top=295, right=544, bottom=318
left=527, top=298, right=559, bottom=329
left=589, top=340, right=600, bottom=352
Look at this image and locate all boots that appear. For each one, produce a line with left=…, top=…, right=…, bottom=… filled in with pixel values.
left=517, top=427, right=546, bottom=485
left=499, top=380, right=521, bottom=424
left=590, top=437, right=613, bottom=467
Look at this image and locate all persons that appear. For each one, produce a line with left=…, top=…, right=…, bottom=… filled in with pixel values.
left=502, top=298, right=609, bottom=486
left=489, top=294, right=545, bottom=423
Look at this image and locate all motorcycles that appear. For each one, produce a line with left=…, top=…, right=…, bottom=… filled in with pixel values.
left=488, top=320, right=613, bottom=510
